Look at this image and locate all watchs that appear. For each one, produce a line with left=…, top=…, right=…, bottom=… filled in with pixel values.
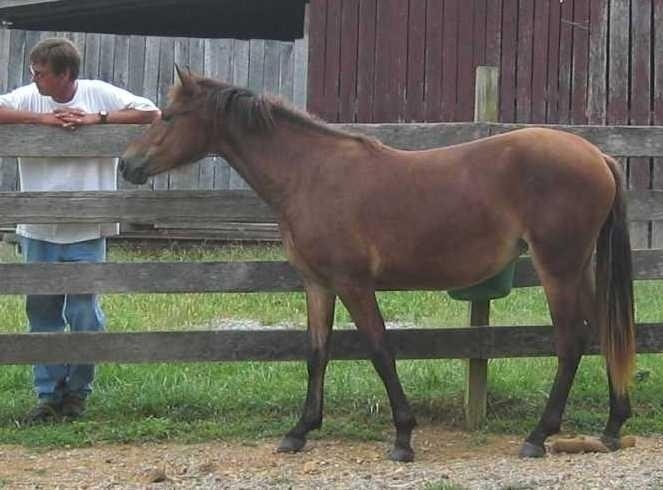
left=99, top=110, right=109, bottom=123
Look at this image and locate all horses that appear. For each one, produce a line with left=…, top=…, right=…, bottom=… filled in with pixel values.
left=115, top=60, right=638, bottom=463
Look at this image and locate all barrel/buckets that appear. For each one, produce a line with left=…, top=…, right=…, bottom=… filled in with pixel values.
left=446, top=262, right=515, bottom=301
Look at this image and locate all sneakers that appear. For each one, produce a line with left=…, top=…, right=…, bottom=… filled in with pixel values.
left=14, top=394, right=88, bottom=430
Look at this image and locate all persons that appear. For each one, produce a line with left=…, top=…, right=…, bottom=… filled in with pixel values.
left=0, top=38, right=162, bottom=421
